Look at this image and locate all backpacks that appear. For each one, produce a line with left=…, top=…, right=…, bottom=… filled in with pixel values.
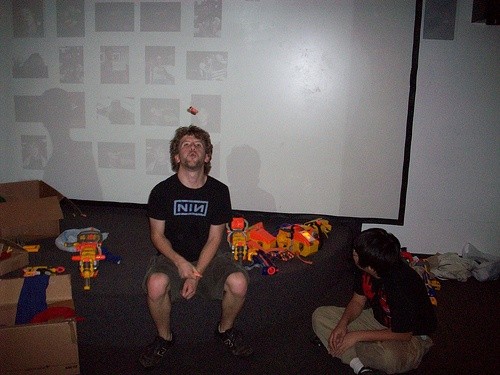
left=427, top=251, right=474, bottom=282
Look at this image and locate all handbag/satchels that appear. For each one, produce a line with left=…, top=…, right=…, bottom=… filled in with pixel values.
left=462, top=241, right=500, bottom=282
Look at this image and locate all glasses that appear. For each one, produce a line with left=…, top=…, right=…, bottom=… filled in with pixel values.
left=181, top=140, right=203, bottom=152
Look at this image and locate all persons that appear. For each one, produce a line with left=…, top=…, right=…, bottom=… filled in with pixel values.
left=134, top=124, right=256, bottom=367
left=312, top=228, right=438, bottom=375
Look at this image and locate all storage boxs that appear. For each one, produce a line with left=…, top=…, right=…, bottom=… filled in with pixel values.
left=0, top=180, right=88, bottom=243
left=0, top=275, right=80, bottom=375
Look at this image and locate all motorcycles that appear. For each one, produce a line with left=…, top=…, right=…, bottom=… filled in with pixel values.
left=226, top=214, right=250, bottom=267
left=71, top=229, right=106, bottom=297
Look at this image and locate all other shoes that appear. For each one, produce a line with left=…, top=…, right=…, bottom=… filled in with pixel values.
left=358, top=366, right=387, bottom=375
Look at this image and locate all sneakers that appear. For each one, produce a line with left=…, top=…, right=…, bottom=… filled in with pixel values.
left=140, top=330, right=177, bottom=366
left=214, top=325, right=255, bottom=358
